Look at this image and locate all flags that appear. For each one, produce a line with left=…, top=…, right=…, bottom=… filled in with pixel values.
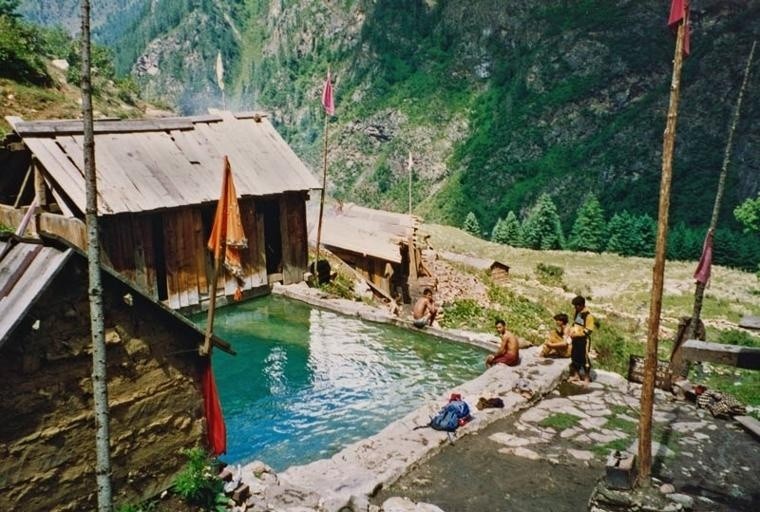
left=208, top=160, right=250, bottom=302
left=322, top=72, right=335, bottom=116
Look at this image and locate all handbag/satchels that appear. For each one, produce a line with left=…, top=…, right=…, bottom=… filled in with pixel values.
left=429, top=401, right=469, bottom=432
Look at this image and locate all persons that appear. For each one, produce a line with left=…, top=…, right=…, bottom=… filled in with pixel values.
left=486, top=320, right=519, bottom=370
left=567, top=295, right=594, bottom=388
left=413, top=288, right=438, bottom=326
left=533, top=313, right=573, bottom=358
